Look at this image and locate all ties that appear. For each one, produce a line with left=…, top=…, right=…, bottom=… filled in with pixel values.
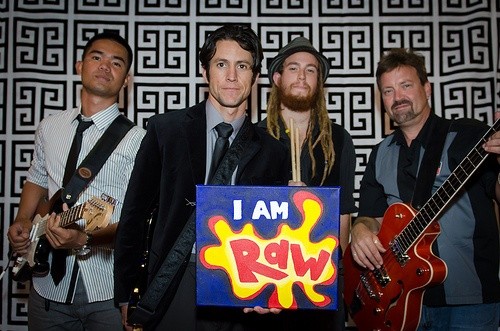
left=50, top=114, right=95, bottom=288
left=206, top=122, right=234, bottom=185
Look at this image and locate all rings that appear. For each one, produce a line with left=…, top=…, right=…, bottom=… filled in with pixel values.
left=375, top=239, right=381, bottom=244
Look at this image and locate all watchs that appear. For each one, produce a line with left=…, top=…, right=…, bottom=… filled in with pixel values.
left=74, top=234, right=93, bottom=256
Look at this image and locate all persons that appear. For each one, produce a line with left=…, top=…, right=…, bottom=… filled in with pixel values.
left=350, top=51, right=500, bottom=331
left=112, top=24, right=282, bottom=331
left=235, top=40, right=357, bottom=331
left=7, top=32, right=148, bottom=331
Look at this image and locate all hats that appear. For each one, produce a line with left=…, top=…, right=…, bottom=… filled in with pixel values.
left=268, top=37, right=331, bottom=86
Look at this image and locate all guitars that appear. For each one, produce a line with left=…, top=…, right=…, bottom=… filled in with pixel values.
left=343, top=118, right=500, bottom=331
left=9, top=188, right=118, bottom=282
